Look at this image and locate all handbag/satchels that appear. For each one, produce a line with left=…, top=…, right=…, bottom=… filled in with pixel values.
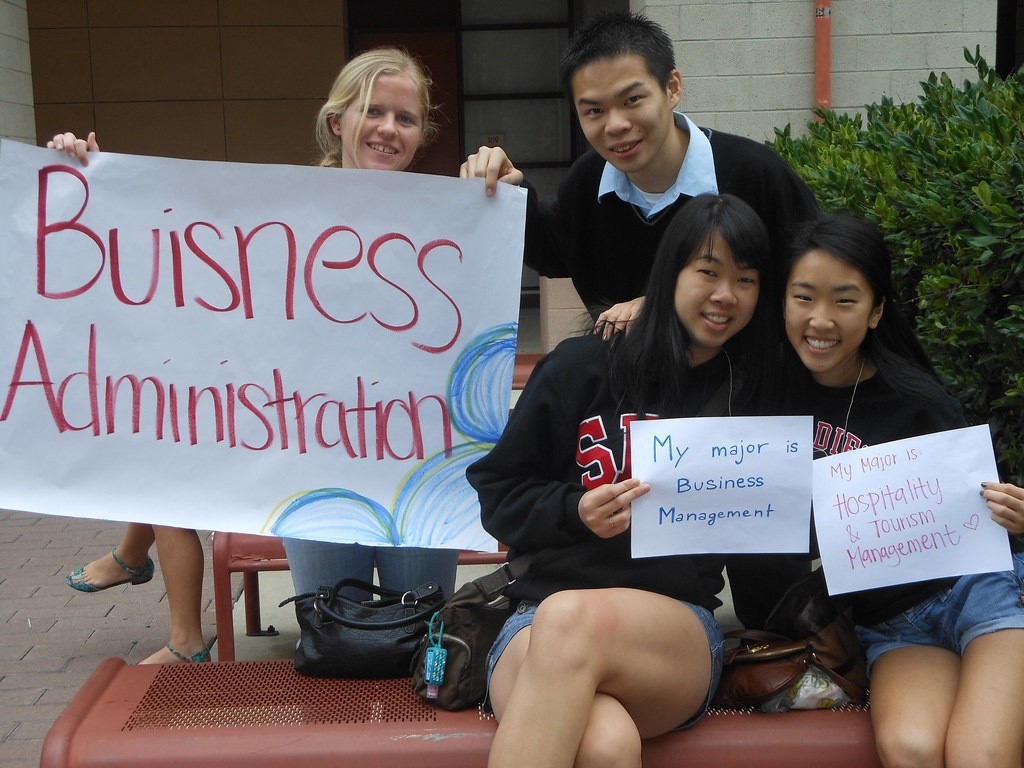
left=279, top=575, right=446, bottom=680
left=710, top=611, right=873, bottom=716
left=413, top=556, right=527, bottom=710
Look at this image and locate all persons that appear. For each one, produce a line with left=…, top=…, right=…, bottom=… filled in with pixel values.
left=46, top=49, right=460, bottom=649
left=458, top=11, right=819, bottom=340
left=64, top=521, right=210, bottom=665
left=465, top=195, right=1024, bottom=768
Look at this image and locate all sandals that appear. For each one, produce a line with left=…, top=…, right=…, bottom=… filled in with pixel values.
left=133, top=644, right=212, bottom=666
left=64, top=547, right=155, bottom=593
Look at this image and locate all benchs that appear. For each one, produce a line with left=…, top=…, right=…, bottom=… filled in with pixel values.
left=211, top=532, right=511, bottom=662
left=41, top=658, right=881, bottom=768
